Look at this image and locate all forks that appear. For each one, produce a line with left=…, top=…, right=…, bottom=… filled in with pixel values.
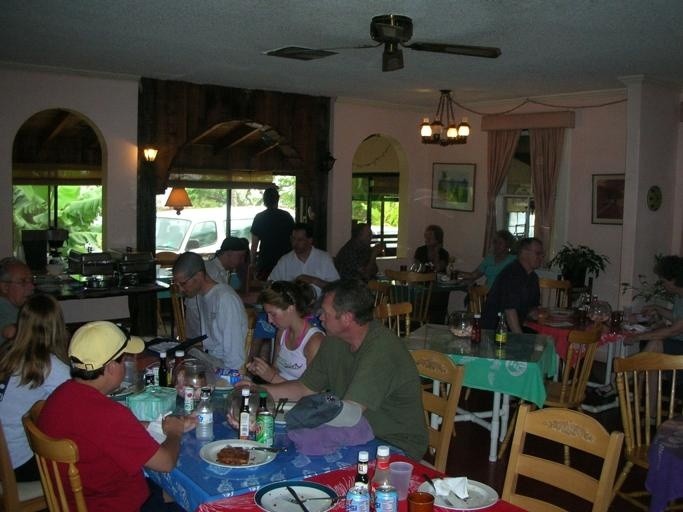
left=281, top=496, right=346, bottom=505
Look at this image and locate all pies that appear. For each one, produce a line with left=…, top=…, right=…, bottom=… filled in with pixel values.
left=217, top=446, right=250, bottom=466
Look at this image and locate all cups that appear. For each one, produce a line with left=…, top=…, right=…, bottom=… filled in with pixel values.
left=87, top=277, right=101, bottom=288
left=400, top=265, right=407, bottom=272
left=608, top=310, right=624, bottom=333
left=450, top=271, right=458, bottom=280
left=388, top=461, right=413, bottom=502
left=407, top=491, right=435, bottom=512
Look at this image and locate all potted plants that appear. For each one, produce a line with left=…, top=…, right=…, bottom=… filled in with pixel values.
left=545, top=240, right=610, bottom=287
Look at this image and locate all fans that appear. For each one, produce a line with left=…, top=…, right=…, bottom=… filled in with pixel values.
left=282, top=14, right=502, bottom=72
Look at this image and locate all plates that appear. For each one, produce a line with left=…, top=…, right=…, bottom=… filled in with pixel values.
left=418, top=477, right=499, bottom=511
left=83, top=287, right=108, bottom=291
left=202, top=384, right=234, bottom=391
left=106, top=381, right=135, bottom=398
left=253, top=480, right=340, bottom=512
left=198, top=439, right=277, bottom=469
left=147, top=342, right=181, bottom=354
left=36, top=284, right=60, bottom=292
left=548, top=306, right=575, bottom=318
left=273, top=402, right=296, bottom=424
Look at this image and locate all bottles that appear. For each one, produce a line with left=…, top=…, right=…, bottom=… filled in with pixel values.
left=239, top=387, right=251, bottom=440
left=494, top=312, right=509, bottom=347
left=446, top=255, right=456, bottom=277
left=256, top=391, right=269, bottom=440
left=122, top=357, right=138, bottom=386
left=354, top=450, right=369, bottom=492
left=370, top=445, right=391, bottom=512
left=592, top=295, right=598, bottom=305
left=195, top=392, right=214, bottom=441
left=583, top=294, right=591, bottom=319
left=171, top=349, right=186, bottom=389
left=159, top=351, right=171, bottom=387
left=471, top=313, right=482, bottom=344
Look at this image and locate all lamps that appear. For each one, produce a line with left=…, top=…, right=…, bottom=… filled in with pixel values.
left=319, top=152, right=337, bottom=175
left=420, top=90, right=471, bottom=146
left=142, top=143, right=159, bottom=180
left=165, top=177, right=192, bottom=215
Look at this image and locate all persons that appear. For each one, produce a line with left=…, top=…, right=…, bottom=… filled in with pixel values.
left=0, top=258, right=35, bottom=359
left=204, top=236, right=248, bottom=288
left=0, top=296, right=75, bottom=481
left=334, top=224, right=386, bottom=292
left=480, top=238, right=545, bottom=335
left=37, top=321, right=186, bottom=512
left=251, top=188, right=295, bottom=280
left=248, top=224, right=340, bottom=361
left=593, top=257, right=683, bottom=427
left=245, top=280, right=325, bottom=392
left=457, top=230, right=517, bottom=304
left=236, top=278, right=429, bottom=462
left=173, top=252, right=249, bottom=373
left=414, top=225, right=449, bottom=325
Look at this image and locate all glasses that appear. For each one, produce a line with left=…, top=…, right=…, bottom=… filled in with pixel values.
left=1, top=276, right=36, bottom=285
left=174, top=269, right=203, bottom=286
left=101, top=325, right=130, bottom=366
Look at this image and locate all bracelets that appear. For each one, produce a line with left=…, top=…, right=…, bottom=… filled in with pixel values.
left=270, top=373, right=275, bottom=384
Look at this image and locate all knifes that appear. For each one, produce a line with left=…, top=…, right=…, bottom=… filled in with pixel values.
left=421, top=472, right=453, bottom=507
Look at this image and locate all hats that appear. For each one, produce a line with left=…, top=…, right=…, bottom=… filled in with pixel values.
left=69, top=321, right=145, bottom=371
left=221, top=237, right=246, bottom=250
left=285, top=392, right=364, bottom=427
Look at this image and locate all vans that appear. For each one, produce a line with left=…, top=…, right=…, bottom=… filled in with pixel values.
left=154, top=206, right=296, bottom=278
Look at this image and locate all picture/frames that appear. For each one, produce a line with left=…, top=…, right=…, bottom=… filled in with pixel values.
left=431, top=162, right=476, bottom=212
left=592, top=174, right=624, bottom=225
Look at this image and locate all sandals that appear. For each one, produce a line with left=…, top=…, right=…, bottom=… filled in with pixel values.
left=595, top=383, right=617, bottom=397
